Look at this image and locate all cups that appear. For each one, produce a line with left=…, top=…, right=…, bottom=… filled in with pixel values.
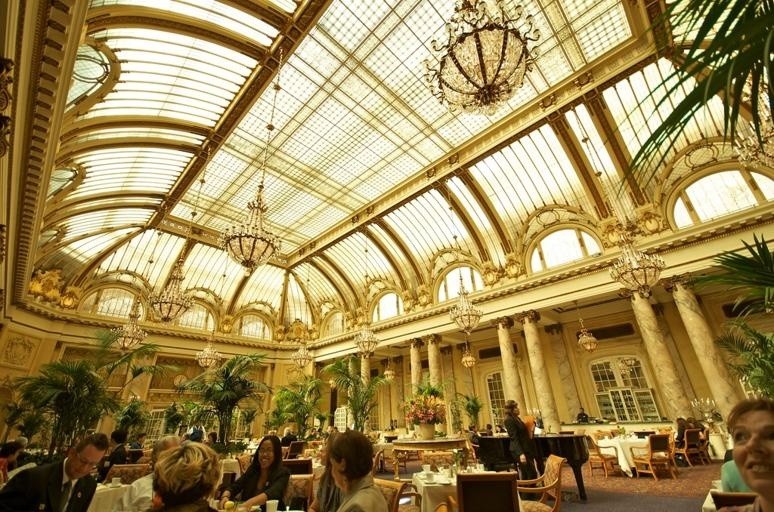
left=265, top=499, right=279, bottom=512
left=111, top=477, right=120, bottom=485
left=419, top=461, right=484, bottom=486
left=211, top=499, right=219, bottom=510
left=224, top=501, right=251, bottom=511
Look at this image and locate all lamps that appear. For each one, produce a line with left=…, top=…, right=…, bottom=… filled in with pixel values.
left=423, top=0, right=541, bottom=115
left=219, top=45, right=285, bottom=277
left=441, top=176, right=484, bottom=335
left=115, top=145, right=233, bottom=369
left=462, top=334, right=476, bottom=369
left=354, top=225, right=396, bottom=382
left=572, top=299, right=599, bottom=352
left=293, top=255, right=314, bottom=369
left=329, top=359, right=337, bottom=389
left=567, top=101, right=667, bottom=298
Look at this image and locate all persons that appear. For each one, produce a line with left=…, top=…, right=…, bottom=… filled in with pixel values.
left=674, top=417, right=692, bottom=462
left=577, top=408, right=588, bottom=423
left=716, top=398, right=774, bottom=512
left=469, top=400, right=544, bottom=500
left=687, top=417, right=706, bottom=461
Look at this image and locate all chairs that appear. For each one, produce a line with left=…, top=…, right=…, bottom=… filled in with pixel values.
left=102, top=428, right=590, bottom=512
left=675, top=430, right=710, bottom=467
left=629, top=433, right=680, bottom=481
left=587, top=428, right=624, bottom=479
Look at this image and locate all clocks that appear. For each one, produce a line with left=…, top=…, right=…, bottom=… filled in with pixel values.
left=174, top=374, right=188, bottom=388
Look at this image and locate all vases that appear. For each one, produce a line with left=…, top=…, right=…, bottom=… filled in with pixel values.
left=415, top=422, right=435, bottom=440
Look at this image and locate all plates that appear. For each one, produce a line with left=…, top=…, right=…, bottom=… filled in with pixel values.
left=108, top=485, right=120, bottom=488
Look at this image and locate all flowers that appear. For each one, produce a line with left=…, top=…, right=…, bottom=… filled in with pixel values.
left=405, top=395, right=448, bottom=424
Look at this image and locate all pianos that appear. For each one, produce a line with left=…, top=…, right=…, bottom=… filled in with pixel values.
left=478, top=435, right=589, bottom=501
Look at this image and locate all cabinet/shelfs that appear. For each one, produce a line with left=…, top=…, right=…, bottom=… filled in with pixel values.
left=594, top=386, right=662, bottom=423
